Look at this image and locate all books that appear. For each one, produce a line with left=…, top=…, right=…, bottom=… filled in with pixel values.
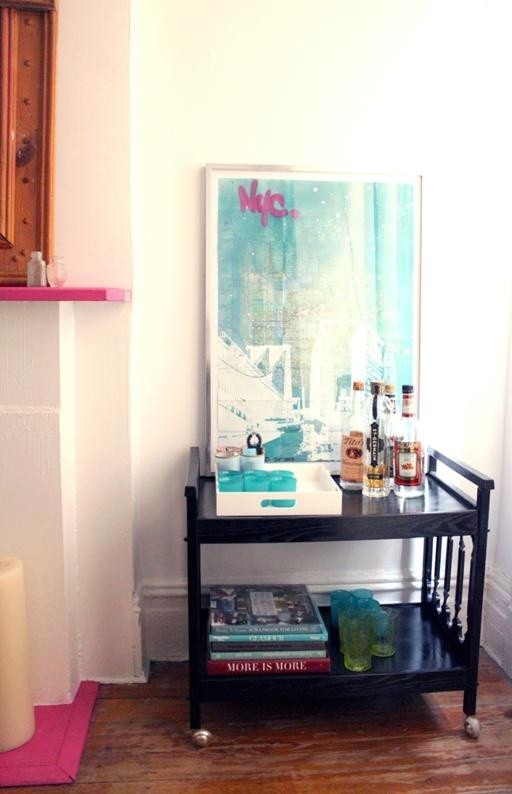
left=206, top=584, right=332, bottom=675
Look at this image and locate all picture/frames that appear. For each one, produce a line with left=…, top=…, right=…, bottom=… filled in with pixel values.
left=1, top=0, right=56, bottom=285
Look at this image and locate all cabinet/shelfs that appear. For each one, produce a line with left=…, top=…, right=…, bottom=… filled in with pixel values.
left=182, top=445, right=493, bottom=736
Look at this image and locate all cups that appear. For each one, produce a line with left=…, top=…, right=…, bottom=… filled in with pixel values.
left=330, top=588, right=399, bottom=671
left=215, top=451, right=297, bottom=508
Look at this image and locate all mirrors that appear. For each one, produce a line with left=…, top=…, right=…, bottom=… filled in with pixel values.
left=204, top=162, right=430, bottom=474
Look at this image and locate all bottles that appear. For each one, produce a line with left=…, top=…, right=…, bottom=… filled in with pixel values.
left=339, top=381, right=425, bottom=498
left=27, top=251, right=68, bottom=286
